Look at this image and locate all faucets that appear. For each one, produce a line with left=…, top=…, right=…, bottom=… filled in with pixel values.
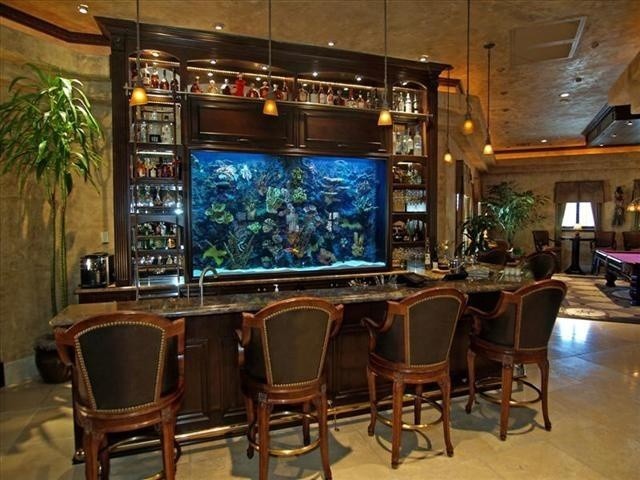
left=198, top=266, right=218, bottom=306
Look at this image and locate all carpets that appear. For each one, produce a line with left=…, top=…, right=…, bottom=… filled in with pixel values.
left=549, top=272, right=640, bottom=326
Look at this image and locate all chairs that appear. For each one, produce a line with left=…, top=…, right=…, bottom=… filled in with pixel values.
left=49, top=309, right=188, bottom=480
left=589, top=230, right=617, bottom=275
left=621, top=231, right=640, bottom=283
left=478, top=227, right=564, bottom=280
left=233, top=295, right=346, bottom=480
left=463, top=278, right=569, bottom=443
left=360, top=285, right=470, bottom=469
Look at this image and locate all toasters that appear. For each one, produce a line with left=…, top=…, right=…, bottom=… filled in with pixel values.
left=80, top=252, right=114, bottom=288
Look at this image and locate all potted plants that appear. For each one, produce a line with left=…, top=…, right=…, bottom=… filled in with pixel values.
left=0, top=57, right=107, bottom=386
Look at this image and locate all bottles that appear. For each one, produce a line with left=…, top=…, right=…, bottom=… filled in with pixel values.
left=394, top=124, right=429, bottom=245
left=188, top=69, right=422, bottom=115
left=128, top=60, right=185, bottom=271
left=424, top=246, right=432, bottom=270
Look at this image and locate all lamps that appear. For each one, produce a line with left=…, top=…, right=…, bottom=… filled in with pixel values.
left=482, top=42, right=497, bottom=157
left=461, top=0, right=476, bottom=135
left=375, top=0, right=393, bottom=128
left=129, top=0, right=149, bottom=108
left=261, top=0, right=280, bottom=119
left=625, top=178, right=640, bottom=212
left=442, top=67, right=453, bottom=164
left=572, top=223, right=583, bottom=237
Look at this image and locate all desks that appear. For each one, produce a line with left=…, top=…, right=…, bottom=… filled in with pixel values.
left=591, top=246, right=640, bottom=306
left=558, top=234, right=595, bottom=276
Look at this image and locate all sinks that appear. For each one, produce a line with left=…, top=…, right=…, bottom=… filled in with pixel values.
left=164, top=302, right=232, bottom=311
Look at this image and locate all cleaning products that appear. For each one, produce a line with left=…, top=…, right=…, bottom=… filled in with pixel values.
left=271, top=283, right=280, bottom=292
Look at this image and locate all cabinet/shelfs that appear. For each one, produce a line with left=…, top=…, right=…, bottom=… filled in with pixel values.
left=94, top=14, right=191, bottom=285
left=383, top=54, right=454, bottom=270
left=178, top=29, right=389, bottom=158
